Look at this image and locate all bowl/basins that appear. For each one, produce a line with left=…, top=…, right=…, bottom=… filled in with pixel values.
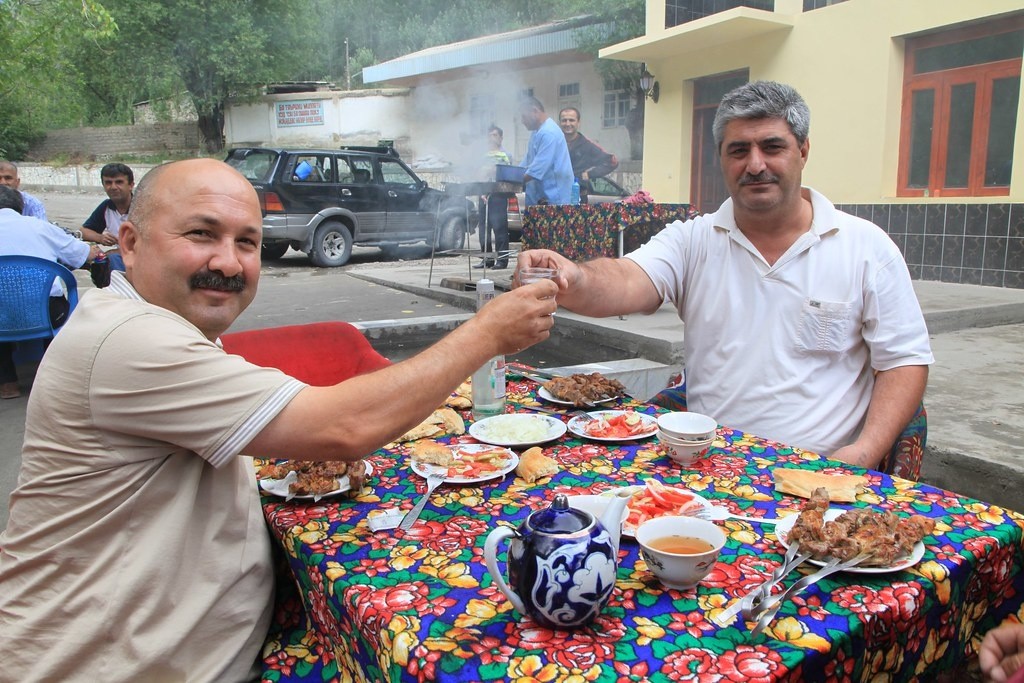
left=565, top=494, right=630, bottom=538
left=656, top=412, right=717, bottom=467
left=634, top=515, right=727, bottom=590
left=496, top=164, right=526, bottom=183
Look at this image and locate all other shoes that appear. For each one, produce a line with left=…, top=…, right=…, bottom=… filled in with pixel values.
left=472, top=259, right=494, bottom=269
left=491, top=259, right=507, bottom=270
left=0, top=381, right=21, bottom=399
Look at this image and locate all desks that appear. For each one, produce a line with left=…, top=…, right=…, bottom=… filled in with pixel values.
left=60, top=237, right=109, bottom=271
left=516, top=203, right=701, bottom=324
left=256, top=361, right=1024, bottom=683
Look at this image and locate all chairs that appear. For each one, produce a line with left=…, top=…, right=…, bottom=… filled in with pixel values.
left=0, top=255, right=80, bottom=396
left=352, top=169, right=372, bottom=182
left=217, top=320, right=393, bottom=388
left=323, top=170, right=331, bottom=180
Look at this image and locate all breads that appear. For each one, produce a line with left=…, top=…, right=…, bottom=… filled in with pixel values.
left=395, top=382, right=473, bottom=443
left=772, top=468, right=870, bottom=503
left=410, top=440, right=454, bottom=466
left=514, top=447, right=559, bottom=483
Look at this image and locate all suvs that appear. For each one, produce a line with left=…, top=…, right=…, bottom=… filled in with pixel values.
left=225, top=147, right=477, bottom=267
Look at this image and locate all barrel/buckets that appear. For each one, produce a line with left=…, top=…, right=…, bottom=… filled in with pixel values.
left=295, top=161, right=313, bottom=181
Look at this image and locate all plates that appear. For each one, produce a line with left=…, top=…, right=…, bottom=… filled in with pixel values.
left=260, top=460, right=372, bottom=498
left=567, top=410, right=658, bottom=440
left=411, top=443, right=519, bottom=483
left=538, top=385, right=620, bottom=405
left=468, top=413, right=567, bottom=446
left=774, top=509, right=926, bottom=575
left=598, top=485, right=710, bottom=538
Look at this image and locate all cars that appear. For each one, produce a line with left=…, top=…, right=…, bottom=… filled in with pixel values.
left=506, top=172, right=633, bottom=242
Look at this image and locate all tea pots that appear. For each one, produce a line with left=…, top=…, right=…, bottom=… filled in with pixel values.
left=483, top=490, right=633, bottom=629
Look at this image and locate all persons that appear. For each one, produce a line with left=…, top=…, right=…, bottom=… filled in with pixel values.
left=517, top=96, right=574, bottom=204
left=0, top=157, right=559, bottom=683
left=513, top=84, right=937, bottom=471
left=0, top=185, right=98, bottom=400
left=471, top=125, right=512, bottom=270
left=0, top=158, right=48, bottom=220
left=81, top=163, right=136, bottom=276
left=558, top=108, right=619, bottom=203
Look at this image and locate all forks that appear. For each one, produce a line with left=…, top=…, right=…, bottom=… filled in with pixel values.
left=681, top=505, right=778, bottom=524
left=399, top=473, right=445, bottom=531
left=520, top=405, right=594, bottom=422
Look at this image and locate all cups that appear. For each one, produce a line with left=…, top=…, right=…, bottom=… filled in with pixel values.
left=518, top=268, right=559, bottom=319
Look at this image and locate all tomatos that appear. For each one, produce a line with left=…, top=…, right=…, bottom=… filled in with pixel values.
left=602, top=481, right=706, bottom=528
left=448, top=446, right=511, bottom=479
left=584, top=411, right=656, bottom=439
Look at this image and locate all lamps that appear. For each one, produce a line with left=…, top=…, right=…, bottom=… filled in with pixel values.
left=638, top=65, right=662, bottom=104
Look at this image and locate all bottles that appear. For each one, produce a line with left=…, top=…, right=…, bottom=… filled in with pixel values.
left=471, top=354, right=507, bottom=421
left=571, top=178, right=580, bottom=206
left=476, top=278, right=494, bottom=313
